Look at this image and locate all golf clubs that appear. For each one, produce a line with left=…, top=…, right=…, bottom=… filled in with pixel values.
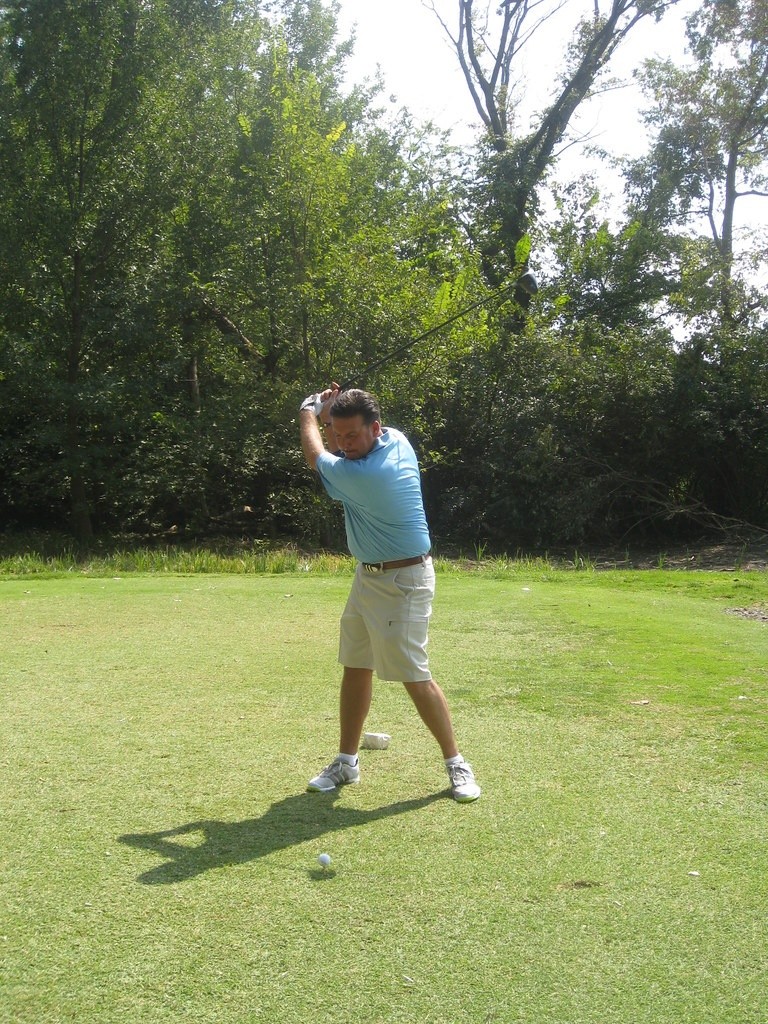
left=321, top=272, right=539, bottom=404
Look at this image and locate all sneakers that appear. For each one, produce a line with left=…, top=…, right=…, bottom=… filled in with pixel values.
left=445, top=756, right=480, bottom=803
left=307, top=757, right=360, bottom=792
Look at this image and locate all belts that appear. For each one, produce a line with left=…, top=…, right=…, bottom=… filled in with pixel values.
left=362, top=551, right=430, bottom=573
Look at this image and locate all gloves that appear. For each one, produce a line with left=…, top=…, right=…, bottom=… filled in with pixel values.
left=299, top=393, right=324, bottom=417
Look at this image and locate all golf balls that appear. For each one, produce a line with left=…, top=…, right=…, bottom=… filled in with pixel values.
left=317, top=853, right=331, bottom=867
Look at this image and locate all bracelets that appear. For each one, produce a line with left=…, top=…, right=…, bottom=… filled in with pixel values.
left=322, top=421, right=333, bottom=428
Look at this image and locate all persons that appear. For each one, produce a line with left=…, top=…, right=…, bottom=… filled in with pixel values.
left=298, top=381, right=482, bottom=804
